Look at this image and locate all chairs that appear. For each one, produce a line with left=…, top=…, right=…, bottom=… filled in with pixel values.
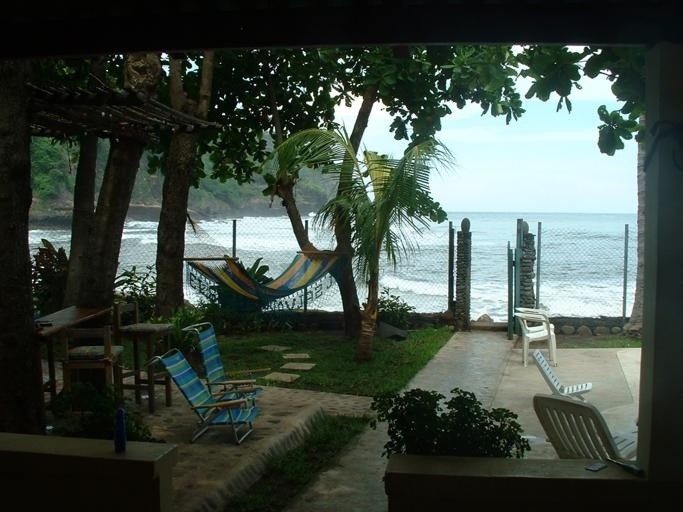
left=514, top=307, right=638, bottom=460
left=65, top=300, right=264, bottom=444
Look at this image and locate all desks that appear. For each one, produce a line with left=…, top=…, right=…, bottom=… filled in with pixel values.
left=35, top=305, right=113, bottom=399
